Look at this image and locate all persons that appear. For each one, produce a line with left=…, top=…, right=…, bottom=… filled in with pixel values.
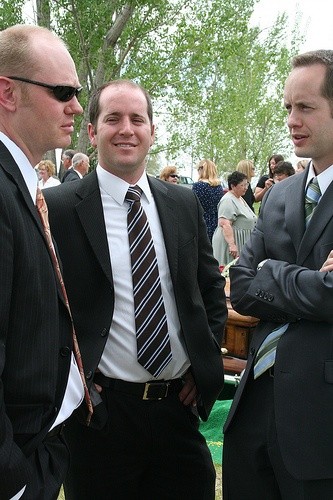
left=0, top=25, right=94, bottom=500
left=43, top=78, right=228, bottom=500
left=157, top=154, right=314, bottom=269
left=222, top=50, right=333, bottom=500
left=35, top=150, right=90, bottom=194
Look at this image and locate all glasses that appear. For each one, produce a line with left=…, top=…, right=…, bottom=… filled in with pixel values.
left=6, top=75, right=84, bottom=102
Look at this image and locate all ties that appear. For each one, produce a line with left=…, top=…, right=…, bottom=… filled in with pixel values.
left=124, top=185, right=173, bottom=380
left=253, top=180, right=321, bottom=377
left=36, top=184, right=94, bottom=427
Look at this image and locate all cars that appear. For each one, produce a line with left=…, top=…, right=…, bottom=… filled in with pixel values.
left=177, top=176, right=195, bottom=189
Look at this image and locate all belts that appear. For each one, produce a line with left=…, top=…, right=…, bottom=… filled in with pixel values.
left=97, top=372, right=185, bottom=401
